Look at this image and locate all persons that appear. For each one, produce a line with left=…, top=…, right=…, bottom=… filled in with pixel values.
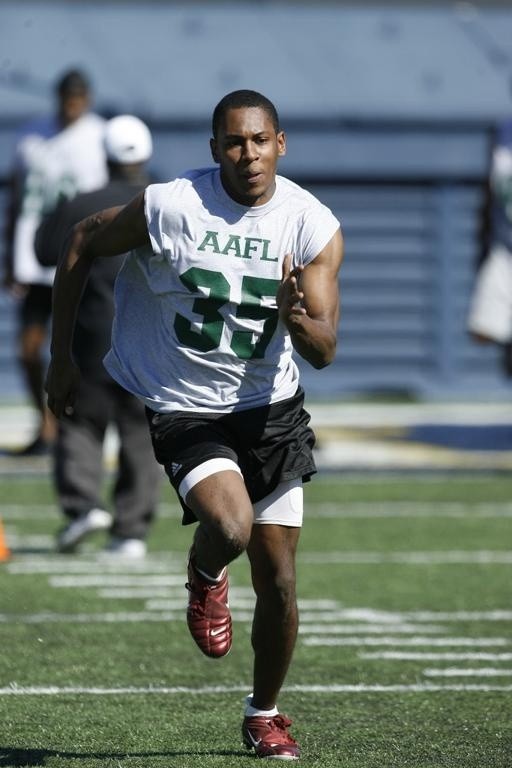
left=44, top=87, right=346, bottom=760
left=32, top=115, right=172, bottom=561
left=468, top=123, right=512, bottom=380
left=1, top=67, right=116, bottom=456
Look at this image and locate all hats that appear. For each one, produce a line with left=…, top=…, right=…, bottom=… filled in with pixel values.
left=103, top=113, right=154, bottom=165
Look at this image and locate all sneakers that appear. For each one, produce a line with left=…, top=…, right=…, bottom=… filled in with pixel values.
left=184, top=544, right=233, bottom=660
left=57, top=506, right=147, bottom=568
left=241, top=691, right=301, bottom=761
